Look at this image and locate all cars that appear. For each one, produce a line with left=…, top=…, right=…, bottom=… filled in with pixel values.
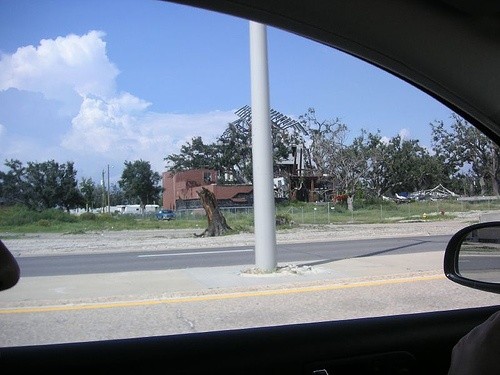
left=155, top=209, right=176, bottom=220
